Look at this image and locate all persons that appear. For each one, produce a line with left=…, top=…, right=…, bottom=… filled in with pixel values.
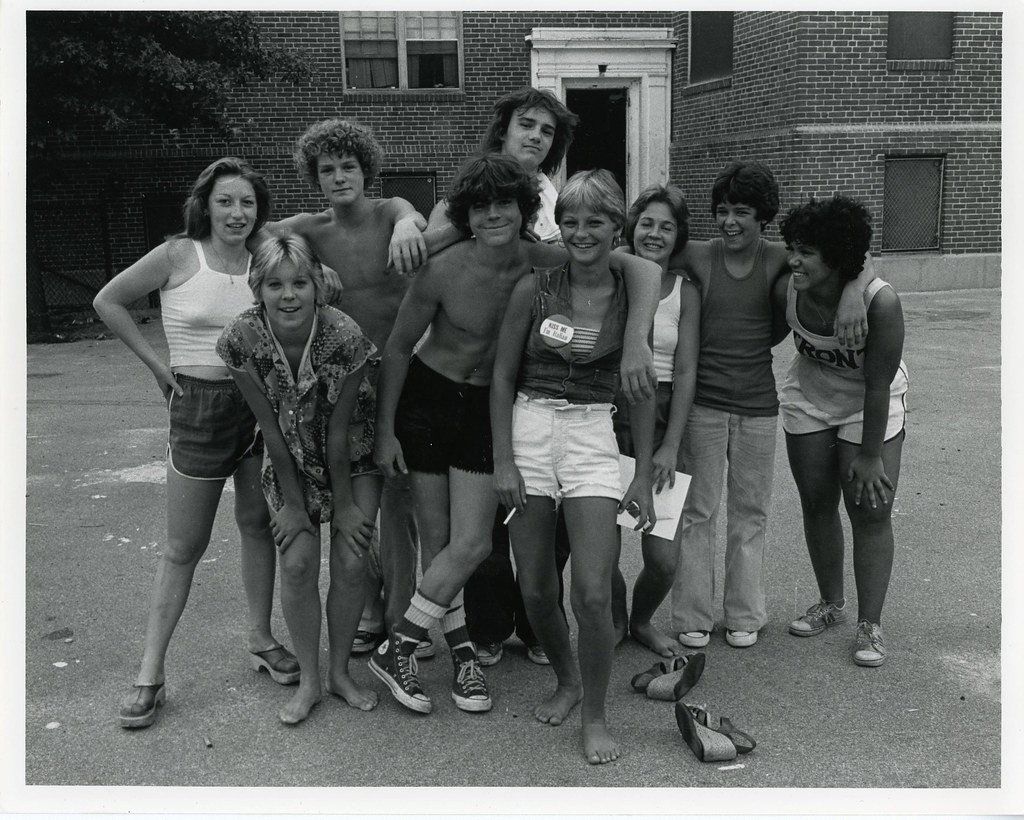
left=92, top=159, right=300, bottom=728
left=368, top=155, right=663, bottom=712
left=613, top=182, right=702, bottom=657
left=614, top=160, right=875, bottom=647
left=491, top=170, right=656, bottom=765
left=421, top=89, right=577, bottom=667
left=214, top=235, right=380, bottom=725
left=249, top=119, right=436, bottom=658
left=772, top=196, right=910, bottom=667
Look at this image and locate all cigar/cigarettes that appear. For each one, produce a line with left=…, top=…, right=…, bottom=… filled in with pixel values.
left=503, top=507, right=516, bottom=525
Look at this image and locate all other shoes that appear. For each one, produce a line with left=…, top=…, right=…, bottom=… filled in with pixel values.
left=726, top=628, right=758, bottom=646
left=414, top=635, right=435, bottom=658
left=680, top=630, right=711, bottom=646
left=351, top=631, right=377, bottom=651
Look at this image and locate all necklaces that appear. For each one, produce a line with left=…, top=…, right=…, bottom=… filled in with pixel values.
left=207, top=237, right=245, bottom=284
left=571, top=283, right=612, bottom=307
left=811, top=297, right=838, bottom=328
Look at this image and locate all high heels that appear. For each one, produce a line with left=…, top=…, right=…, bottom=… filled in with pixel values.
left=250, top=645, right=301, bottom=684
left=631, top=652, right=706, bottom=702
left=120, top=682, right=166, bottom=728
left=674, top=701, right=756, bottom=762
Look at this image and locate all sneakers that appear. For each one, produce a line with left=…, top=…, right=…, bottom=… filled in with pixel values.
left=789, top=598, right=848, bottom=636
left=450, top=646, right=492, bottom=711
left=852, top=619, right=887, bottom=666
left=474, top=637, right=503, bottom=665
left=367, top=624, right=431, bottom=713
left=527, top=644, right=551, bottom=664
